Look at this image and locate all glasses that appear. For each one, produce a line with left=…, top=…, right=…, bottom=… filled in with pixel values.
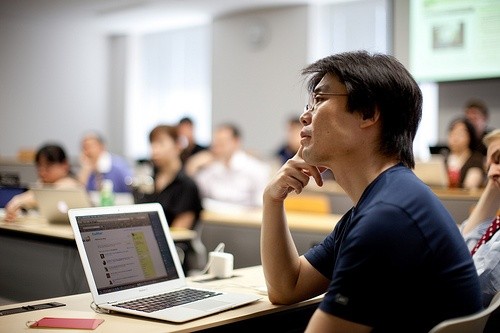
left=305, top=90, right=348, bottom=111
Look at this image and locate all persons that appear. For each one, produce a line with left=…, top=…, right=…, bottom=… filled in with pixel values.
left=429, top=102, right=500, bottom=300
left=3, top=118, right=336, bottom=259
left=261, top=51, right=482, bottom=333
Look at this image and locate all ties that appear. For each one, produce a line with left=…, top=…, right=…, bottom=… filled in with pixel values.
left=470, top=216, right=500, bottom=257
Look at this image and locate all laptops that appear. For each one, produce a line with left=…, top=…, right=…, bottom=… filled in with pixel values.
left=29, top=188, right=94, bottom=225
left=67, top=202, right=263, bottom=323
